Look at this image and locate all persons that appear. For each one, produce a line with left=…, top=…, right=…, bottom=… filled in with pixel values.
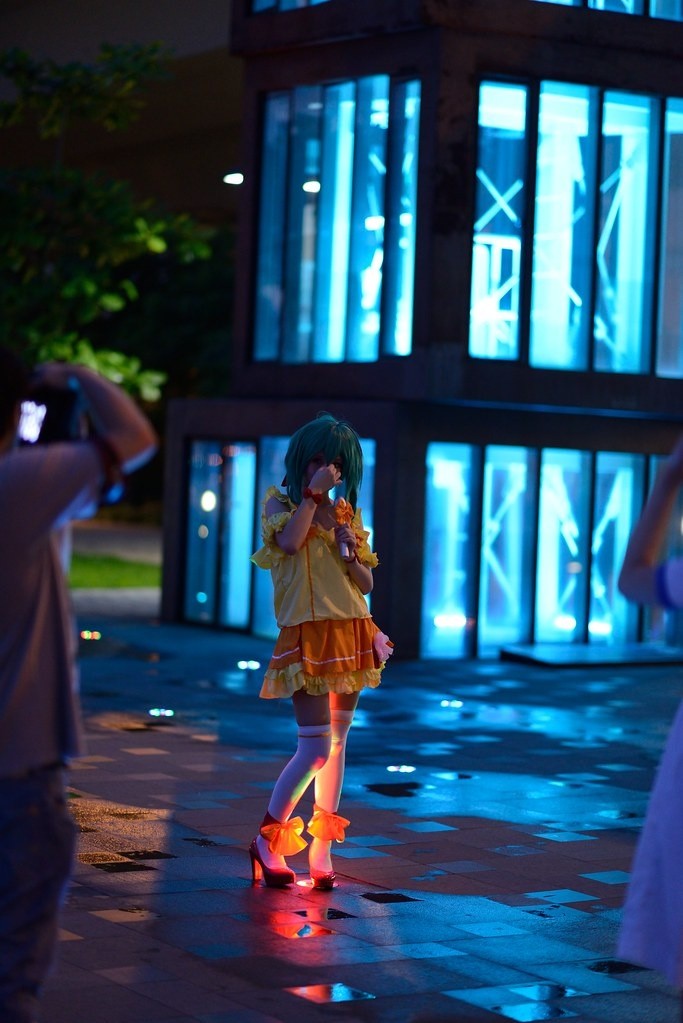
left=617, top=436, right=682, bottom=996
left=250, top=411, right=396, bottom=889
left=0, top=327, right=157, bottom=1023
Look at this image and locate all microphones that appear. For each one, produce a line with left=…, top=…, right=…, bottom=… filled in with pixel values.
left=333, top=496, right=354, bottom=560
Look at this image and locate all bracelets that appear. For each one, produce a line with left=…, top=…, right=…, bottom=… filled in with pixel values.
left=344, top=548, right=362, bottom=565
left=303, top=488, right=323, bottom=505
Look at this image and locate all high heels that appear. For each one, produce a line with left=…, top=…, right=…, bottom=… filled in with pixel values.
left=307, top=846, right=335, bottom=890
left=249, top=835, right=296, bottom=886
